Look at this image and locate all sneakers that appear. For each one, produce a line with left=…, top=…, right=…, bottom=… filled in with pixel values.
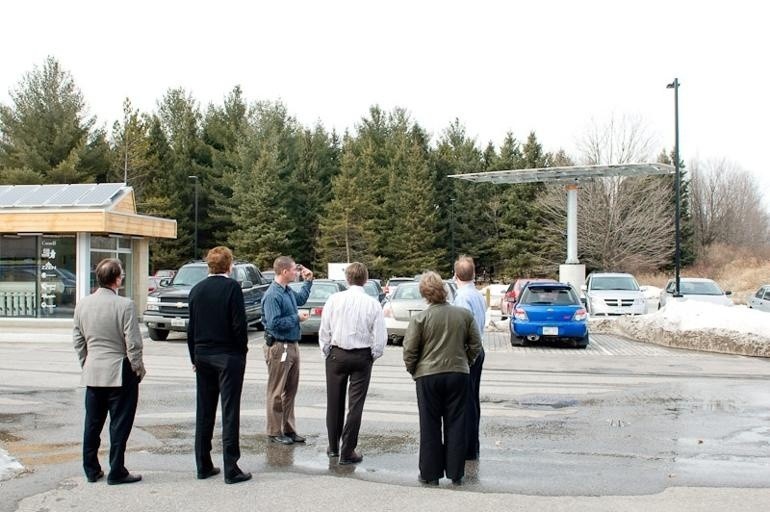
left=417, top=473, right=440, bottom=487
left=451, top=475, right=465, bottom=486
left=465, top=451, right=480, bottom=463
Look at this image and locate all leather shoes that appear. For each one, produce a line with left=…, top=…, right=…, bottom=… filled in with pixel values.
left=326, top=445, right=340, bottom=459
left=107, top=473, right=143, bottom=486
left=284, top=431, right=307, bottom=442
left=269, top=435, right=294, bottom=446
left=339, top=453, right=364, bottom=465
left=224, top=471, right=253, bottom=484
left=197, top=467, right=221, bottom=480
left=87, top=470, right=105, bottom=484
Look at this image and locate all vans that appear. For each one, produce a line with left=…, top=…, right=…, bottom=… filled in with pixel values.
left=0, top=263, right=76, bottom=304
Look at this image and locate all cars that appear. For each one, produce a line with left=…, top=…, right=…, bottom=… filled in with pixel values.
left=259, top=262, right=460, bottom=345
left=478, top=277, right=590, bottom=353
left=657, top=278, right=734, bottom=311
left=746, top=284, right=770, bottom=313
left=91, top=271, right=101, bottom=295
left=148, top=276, right=163, bottom=295
left=155, top=270, right=176, bottom=278
left=580, top=272, right=647, bottom=318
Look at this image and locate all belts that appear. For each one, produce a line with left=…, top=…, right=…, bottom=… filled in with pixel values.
left=273, top=339, right=301, bottom=344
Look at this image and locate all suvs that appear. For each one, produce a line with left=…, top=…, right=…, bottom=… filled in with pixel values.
left=142, top=263, right=271, bottom=340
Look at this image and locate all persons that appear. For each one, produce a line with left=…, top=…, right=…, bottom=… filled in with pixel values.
left=259, top=256, right=314, bottom=443
left=70, top=259, right=146, bottom=485
left=317, top=262, right=389, bottom=464
left=449, top=255, right=486, bottom=459
left=403, top=271, right=484, bottom=485
left=186, top=246, right=252, bottom=483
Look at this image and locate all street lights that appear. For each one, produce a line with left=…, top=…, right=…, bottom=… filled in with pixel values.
left=188, top=175, right=198, bottom=259
left=665, top=79, right=685, bottom=298
left=449, top=197, right=456, bottom=277
